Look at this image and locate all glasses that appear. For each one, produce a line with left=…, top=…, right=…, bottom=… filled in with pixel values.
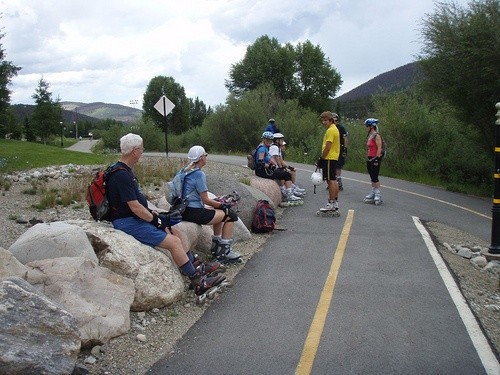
left=135, top=147, right=145, bottom=152
left=333, top=120, right=338, bottom=122
left=366, top=125, right=371, bottom=128
left=201, top=153, right=207, bottom=160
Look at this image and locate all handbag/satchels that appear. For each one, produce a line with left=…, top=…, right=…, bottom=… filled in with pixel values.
left=219, top=190, right=241, bottom=213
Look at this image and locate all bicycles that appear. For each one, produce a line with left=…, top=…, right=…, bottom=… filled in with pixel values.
left=89, top=136, right=93, bottom=142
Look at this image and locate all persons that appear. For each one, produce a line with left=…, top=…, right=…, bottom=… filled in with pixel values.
left=181, top=146, right=243, bottom=269
left=315, top=112, right=340, bottom=217
left=264, top=119, right=276, bottom=134
left=326, top=113, right=347, bottom=191
left=106, top=133, right=234, bottom=307
left=269, top=133, right=306, bottom=195
left=364, top=119, right=383, bottom=205
left=254, top=132, right=304, bottom=207
left=88, top=131, right=93, bottom=140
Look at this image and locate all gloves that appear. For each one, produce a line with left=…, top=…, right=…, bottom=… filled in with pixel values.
left=316, top=156, right=325, bottom=169
left=371, top=158, right=379, bottom=169
left=269, top=163, right=296, bottom=174
left=214, top=198, right=235, bottom=211
left=341, top=148, right=347, bottom=159
left=149, top=212, right=182, bottom=229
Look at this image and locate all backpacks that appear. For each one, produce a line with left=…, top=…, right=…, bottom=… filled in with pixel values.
left=162, top=166, right=200, bottom=208
left=374, top=133, right=386, bottom=160
left=86, top=166, right=137, bottom=222
left=247, top=145, right=264, bottom=170
left=251, top=199, right=287, bottom=234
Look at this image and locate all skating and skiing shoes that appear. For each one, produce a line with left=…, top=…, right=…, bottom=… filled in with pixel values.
left=190, top=272, right=234, bottom=308
left=315, top=201, right=340, bottom=217
left=374, top=192, right=383, bottom=205
left=279, top=185, right=306, bottom=207
left=362, top=190, right=375, bottom=204
left=188, top=254, right=226, bottom=291
left=211, top=236, right=243, bottom=269
left=326, top=177, right=343, bottom=190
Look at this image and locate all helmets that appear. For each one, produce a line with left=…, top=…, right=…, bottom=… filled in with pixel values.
left=364, top=118, right=379, bottom=125
left=263, top=118, right=286, bottom=146
left=311, top=172, right=325, bottom=186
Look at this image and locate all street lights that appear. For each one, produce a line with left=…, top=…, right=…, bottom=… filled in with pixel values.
left=60, top=122, right=64, bottom=146
left=73, top=120, right=76, bottom=139
